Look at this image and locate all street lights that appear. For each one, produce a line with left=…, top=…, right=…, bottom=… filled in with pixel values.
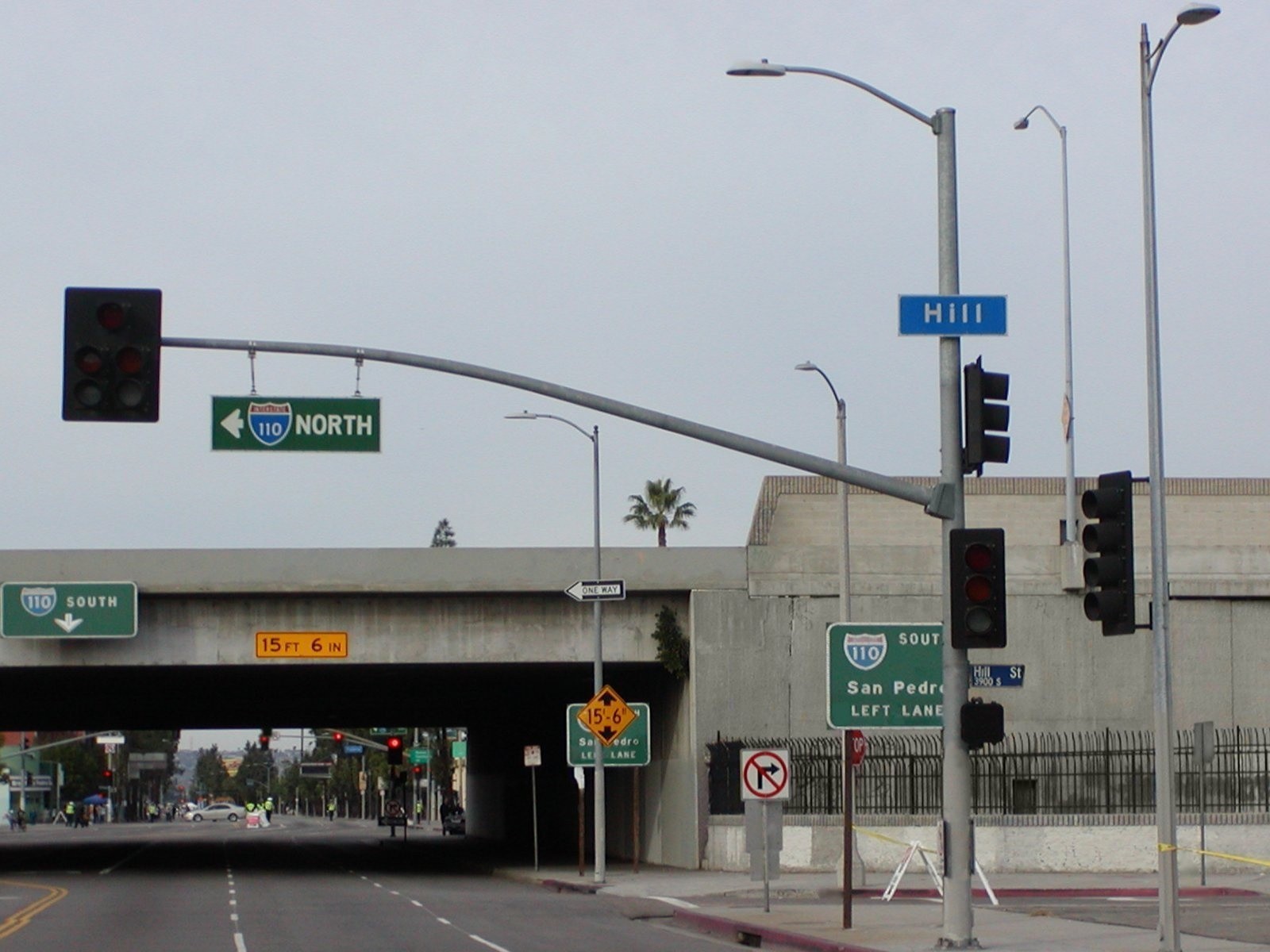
left=1012, top=104, right=1078, bottom=543
left=721, top=56, right=976, bottom=952
left=504, top=408, right=606, bottom=884
left=794, top=360, right=853, bottom=928
left=1136, top=1, right=1227, bottom=952
left=253, top=762, right=279, bottom=796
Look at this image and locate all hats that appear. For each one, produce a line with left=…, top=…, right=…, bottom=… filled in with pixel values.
left=267, top=797, right=273, bottom=801
left=69, top=802, right=74, bottom=805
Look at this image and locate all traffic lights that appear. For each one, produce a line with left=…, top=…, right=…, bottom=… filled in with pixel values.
left=947, top=527, right=1007, bottom=649
left=1083, top=470, right=1137, bottom=637
left=261, top=734, right=269, bottom=751
left=61, top=287, right=160, bottom=424
left=333, top=732, right=343, bottom=748
left=102, top=769, right=112, bottom=786
left=26, top=771, right=33, bottom=788
left=963, top=353, right=1011, bottom=478
left=415, top=766, right=422, bottom=781
left=387, top=735, right=404, bottom=765
left=25, top=736, right=29, bottom=750
left=960, top=699, right=1004, bottom=745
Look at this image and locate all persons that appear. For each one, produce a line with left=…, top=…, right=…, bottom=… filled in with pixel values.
left=415, top=801, right=424, bottom=824
left=143, top=798, right=160, bottom=823
left=73, top=802, right=86, bottom=827
left=3, top=808, right=26, bottom=830
left=246, top=798, right=255, bottom=812
left=327, top=798, right=336, bottom=821
left=18, top=808, right=27, bottom=832
left=64, top=801, right=75, bottom=827
left=99, top=803, right=106, bottom=824
left=440, top=795, right=455, bottom=837
left=165, top=802, right=190, bottom=822
left=93, top=805, right=98, bottom=823
left=256, top=797, right=265, bottom=810
left=265, top=797, right=273, bottom=822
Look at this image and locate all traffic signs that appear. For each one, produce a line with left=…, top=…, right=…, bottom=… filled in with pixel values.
left=0, top=581, right=138, bottom=639
left=210, top=394, right=382, bottom=452
left=564, top=576, right=626, bottom=602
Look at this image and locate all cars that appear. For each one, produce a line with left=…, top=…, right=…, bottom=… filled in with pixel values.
left=183, top=801, right=247, bottom=823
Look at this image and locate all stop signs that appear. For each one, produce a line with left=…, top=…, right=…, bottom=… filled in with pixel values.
left=852, top=730, right=868, bottom=767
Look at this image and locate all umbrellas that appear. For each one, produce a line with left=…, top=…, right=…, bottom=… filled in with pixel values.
left=83, top=795, right=107, bottom=805
